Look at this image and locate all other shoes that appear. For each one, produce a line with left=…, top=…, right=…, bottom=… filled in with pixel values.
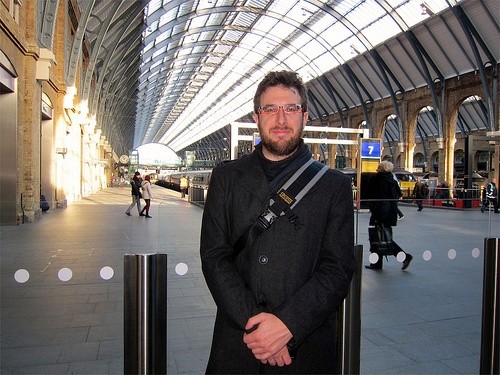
left=139, top=212, right=146, bottom=216
left=125, top=212, right=132, bottom=216
left=145, top=215, right=152, bottom=218
left=402, top=253, right=413, bottom=270
left=399, top=215, right=404, bottom=219
left=365, top=259, right=383, bottom=270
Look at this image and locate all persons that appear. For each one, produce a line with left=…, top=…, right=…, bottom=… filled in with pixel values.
left=125, top=172, right=153, bottom=218
left=179, top=176, right=188, bottom=198
left=398, top=176, right=500, bottom=221
left=200, top=70, right=355, bottom=375
left=364, top=161, right=413, bottom=271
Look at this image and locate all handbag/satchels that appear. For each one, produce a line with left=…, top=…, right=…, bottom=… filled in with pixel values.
left=139, top=192, right=142, bottom=198
left=368, top=220, right=395, bottom=262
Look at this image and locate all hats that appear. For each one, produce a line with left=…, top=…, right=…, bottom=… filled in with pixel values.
left=145, top=176, right=150, bottom=181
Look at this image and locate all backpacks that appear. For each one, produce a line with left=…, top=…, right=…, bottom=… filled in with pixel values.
left=423, top=185, right=426, bottom=195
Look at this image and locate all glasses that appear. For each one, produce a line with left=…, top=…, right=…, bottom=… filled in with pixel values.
left=257, top=104, right=304, bottom=115
